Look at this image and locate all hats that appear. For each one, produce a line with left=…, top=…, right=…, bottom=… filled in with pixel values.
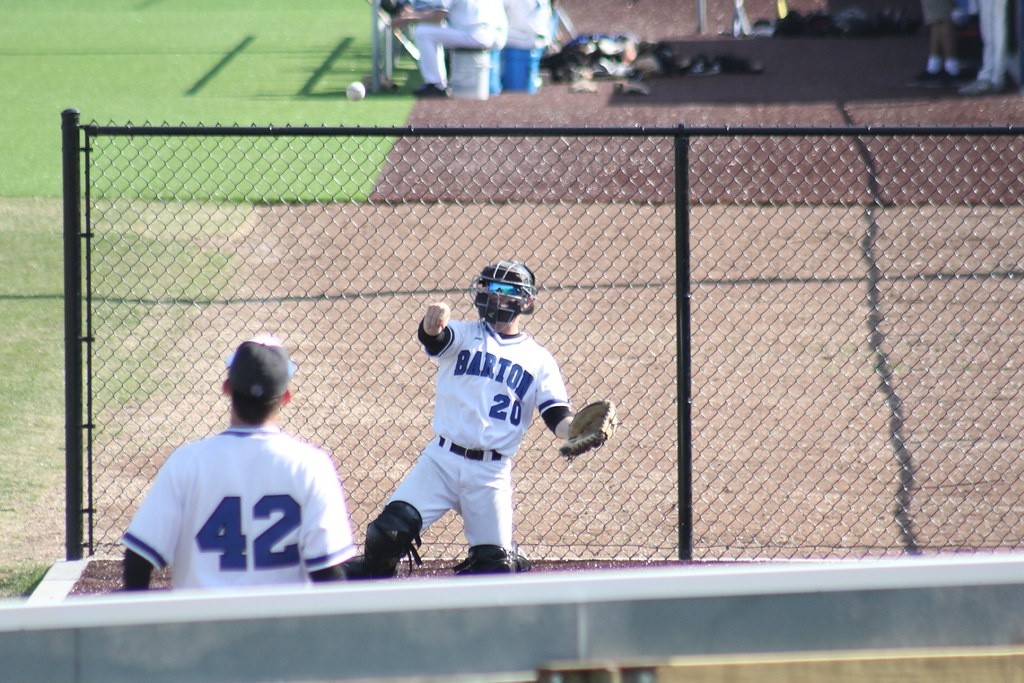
left=228, top=335, right=295, bottom=400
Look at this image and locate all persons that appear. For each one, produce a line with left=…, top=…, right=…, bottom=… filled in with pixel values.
left=500, top=0, right=555, bottom=52
left=365, top=252, right=616, bottom=579
left=483, top=0, right=509, bottom=53
left=116, top=332, right=398, bottom=594
left=903, top=0, right=972, bottom=90
left=391, top=0, right=510, bottom=98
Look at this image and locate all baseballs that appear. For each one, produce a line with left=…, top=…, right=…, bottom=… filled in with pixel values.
left=347, top=81, right=365, bottom=101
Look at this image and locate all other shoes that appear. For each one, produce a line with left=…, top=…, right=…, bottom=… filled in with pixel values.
left=955, top=80, right=1001, bottom=99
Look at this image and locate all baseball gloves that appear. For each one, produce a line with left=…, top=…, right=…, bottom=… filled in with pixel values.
left=559, top=400, right=618, bottom=455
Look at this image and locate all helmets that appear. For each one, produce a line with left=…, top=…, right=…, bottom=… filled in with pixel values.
left=478, top=261, right=534, bottom=323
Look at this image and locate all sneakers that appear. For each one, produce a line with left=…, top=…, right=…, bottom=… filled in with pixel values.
left=555, top=29, right=761, bottom=100
left=904, top=69, right=962, bottom=90
left=414, top=83, right=452, bottom=99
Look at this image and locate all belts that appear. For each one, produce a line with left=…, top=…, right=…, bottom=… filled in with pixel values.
left=439, top=436, right=502, bottom=463
